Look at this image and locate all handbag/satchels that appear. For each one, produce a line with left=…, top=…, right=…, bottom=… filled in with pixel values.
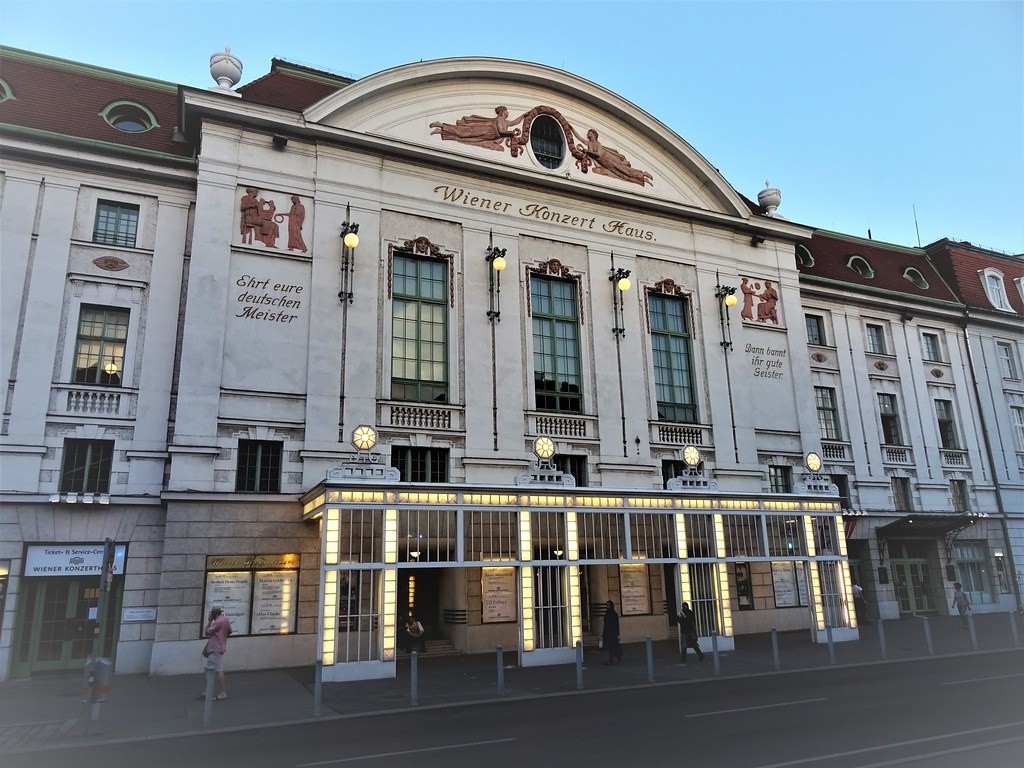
left=202, top=642, right=210, bottom=658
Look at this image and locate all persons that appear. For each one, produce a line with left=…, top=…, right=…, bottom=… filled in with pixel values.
left=603, top=600, right=624, bottom=665
left=851, top=578, right=868, bottom=627
left=952, top=582, right=972, bottom=630
left=196, top=606, right=233, bottom=701
left=405, top=616, right=428, bottom=653
left=676, top=602, right=706, bottom=662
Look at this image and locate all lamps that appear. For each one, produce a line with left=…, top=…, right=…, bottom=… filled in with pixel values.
left=272, top=134, right=287, bottom=147
left=348, top=424, right=381, bottom=463
left=66, top=492, right=78, bottom=503
left=338, top=201, right=360, bottom=304
left=715, top=269, right=737, bottom=352
left=533, top=435, right=556, bottom=471
left=900, top=312, right=913, bottom=323
left=98, top=494, right=111, bottom=504
left=681, top=444, right=703, bottom=476
left=49, top=492, right=61, bottom=503
left=485, top=228, right=507, bottom=323
left=751, top=235, right=766, bottom=247
left=608, top=249, right=631, bottom=338
left=805, top=452, right=822, bottom=481
left=83, top=492, right=94, bottom=503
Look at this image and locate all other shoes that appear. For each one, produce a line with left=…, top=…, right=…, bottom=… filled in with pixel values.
left=212, top=694, right=227, bottom=701
left=963, top=625, right=969, bottom=629
left=201, top=692, right=206, bottom=697
left=602, top=661, right=613, bottom=665
left=420, top=649, right=427, bottom=653
left=678, top=656, right=688, bottom=662
left=407, top=649, right=411, bottom=654
left=701, top=655, right=706, bottom=661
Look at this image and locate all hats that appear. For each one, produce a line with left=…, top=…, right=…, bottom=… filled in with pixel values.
left=209, top=605, right=224, bottom=613
left=954, top=583, right=961, bottom=587
